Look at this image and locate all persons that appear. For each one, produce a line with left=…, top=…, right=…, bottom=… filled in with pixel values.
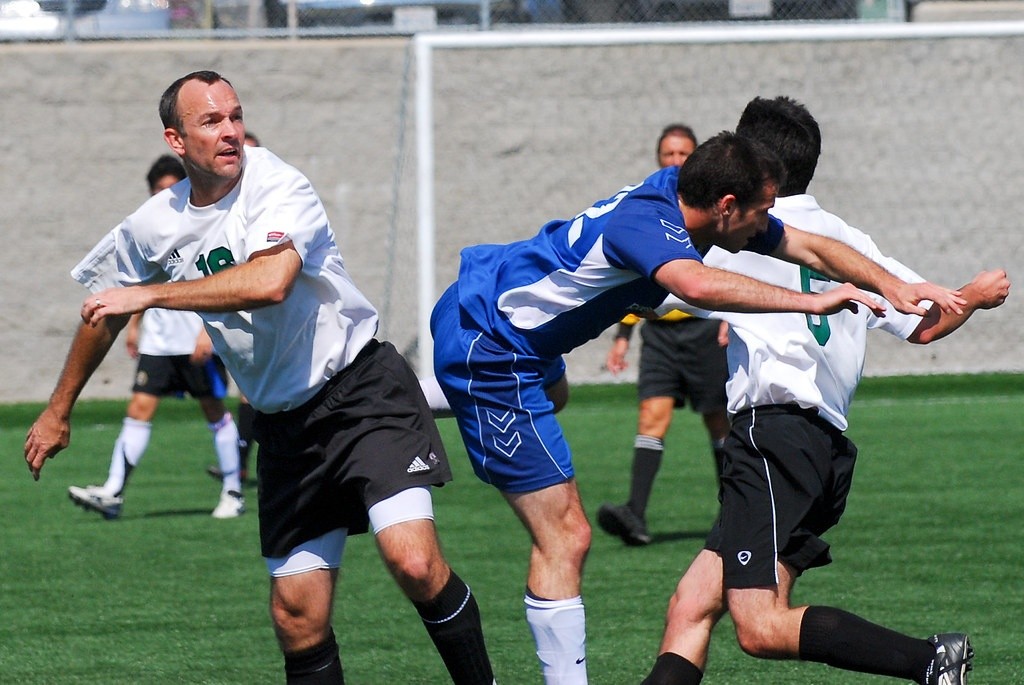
left=595, top=123, right=727, bottom=547
left=430, top=132, right=967, bottom=685
left=637, top=95, right=1011, bottom=685
left=22, top=70, right=493, bottom=685
left=65, top=154, right=246, bottom=519
left=207, top=133, right=263, bottom=481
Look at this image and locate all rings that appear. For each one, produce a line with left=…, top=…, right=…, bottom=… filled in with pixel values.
left=96, top=298, right=100, bottom=307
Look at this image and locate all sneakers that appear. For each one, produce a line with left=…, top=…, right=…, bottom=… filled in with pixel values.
left=207, top=462, right=246, bottom=486
left=68, top=485, right=123, bottom=519
left=922, top=633, right=974, bottom=685
left=598, top=502, right=650, bottom=545
left=212, top=488, right=246, bottom=519
